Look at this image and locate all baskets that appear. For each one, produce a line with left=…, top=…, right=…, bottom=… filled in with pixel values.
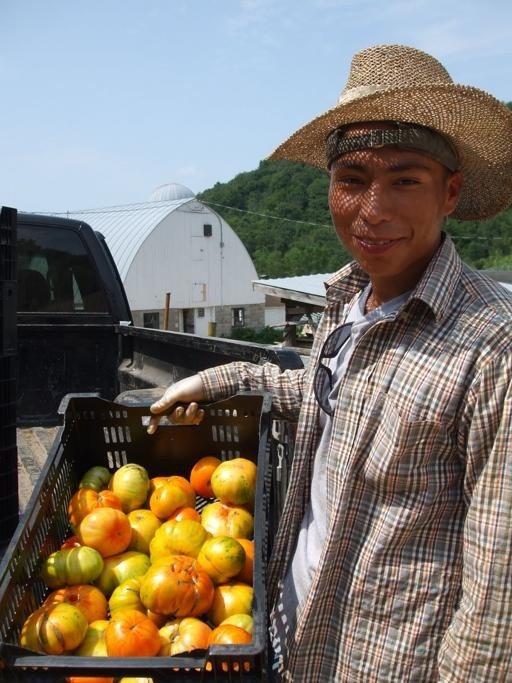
left=0, top=389, right=280, bottom=682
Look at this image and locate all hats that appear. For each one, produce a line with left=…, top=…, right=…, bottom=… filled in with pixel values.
left=265, top=43, right=512, bottom=221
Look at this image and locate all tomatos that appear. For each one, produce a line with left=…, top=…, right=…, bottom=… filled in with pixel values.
left=19, top=457, right=256, bottom=682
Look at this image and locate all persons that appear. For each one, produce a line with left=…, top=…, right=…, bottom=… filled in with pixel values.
left=147, top=45, right=512, bottom=683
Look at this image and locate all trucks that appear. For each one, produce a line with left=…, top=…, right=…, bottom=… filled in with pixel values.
left=1, top=206, right=305, bottom=557
